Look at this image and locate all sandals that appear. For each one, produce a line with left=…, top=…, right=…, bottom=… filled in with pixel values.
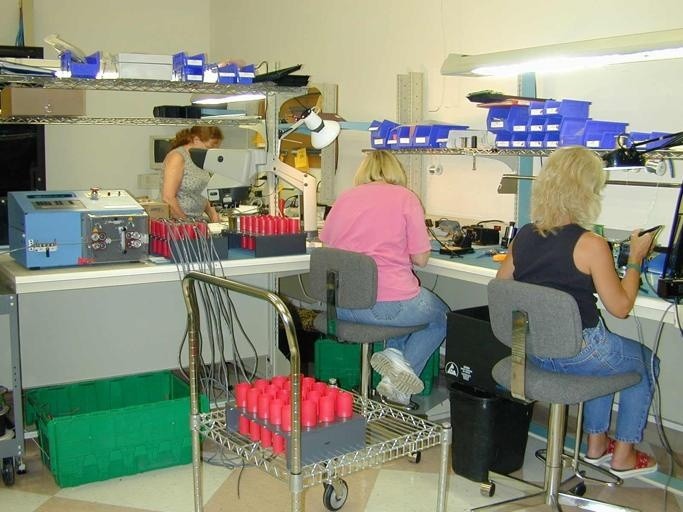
left=583, top=437, right=617, bottom=466
left=609, top=449, right=658, bottom=479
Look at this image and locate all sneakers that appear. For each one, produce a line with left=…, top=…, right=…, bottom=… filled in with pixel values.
left=376, top=377, right=411, bottom=406
left=369, top=349, right=425, bottom=395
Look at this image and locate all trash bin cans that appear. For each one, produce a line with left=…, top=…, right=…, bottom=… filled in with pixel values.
left=445, top=307, right=534, bottom=477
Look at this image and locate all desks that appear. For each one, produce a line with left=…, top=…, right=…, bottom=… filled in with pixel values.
left=1, top=239, right=683, bottom=429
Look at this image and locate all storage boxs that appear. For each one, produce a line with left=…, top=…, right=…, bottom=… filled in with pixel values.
left=21, top=372, right=208, bottom=485
left=315, top=304, right=512, bottom=399
left=115, top=53, right=174, bottom=79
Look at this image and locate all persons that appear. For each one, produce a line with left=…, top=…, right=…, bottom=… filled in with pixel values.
left=157, top=128, right=226, bottom=221
left=316, top=148, right=450, bottom=408
left=493, top=146, right=660, bottom=479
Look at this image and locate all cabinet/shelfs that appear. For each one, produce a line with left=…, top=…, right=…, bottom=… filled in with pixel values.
left=0, top=75, right=307, bottom=129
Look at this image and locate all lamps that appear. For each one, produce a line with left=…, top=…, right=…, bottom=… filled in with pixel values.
left=601, top=130, right=683, bottom=279
left=274, top=110, right=341, bottom=159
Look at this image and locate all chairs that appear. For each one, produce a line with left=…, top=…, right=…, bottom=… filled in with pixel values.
left=472, top=274, right=642, bottom=511
left=307, top=248, right=428, bottom=462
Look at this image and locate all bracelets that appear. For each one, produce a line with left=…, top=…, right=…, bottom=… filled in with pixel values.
left=622, top=264, right=645, bottom=276
